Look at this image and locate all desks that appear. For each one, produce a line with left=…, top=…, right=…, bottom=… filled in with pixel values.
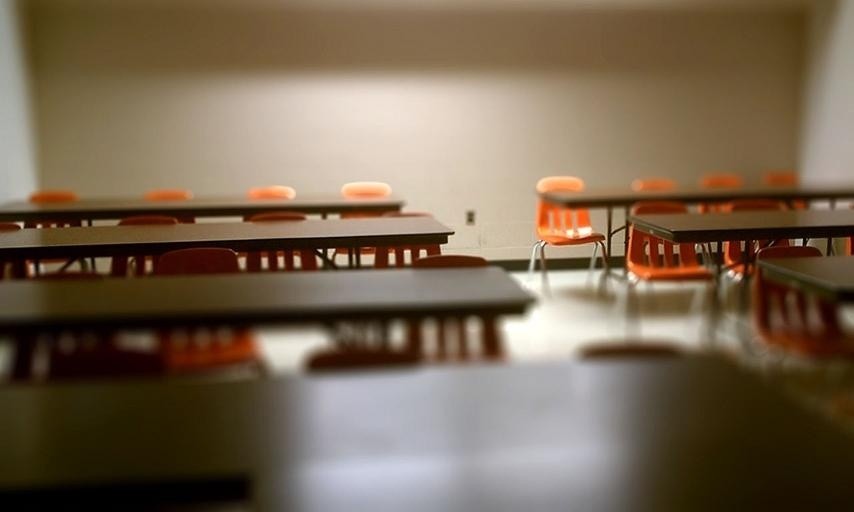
left=0, top=355, right=852, bottom=510
